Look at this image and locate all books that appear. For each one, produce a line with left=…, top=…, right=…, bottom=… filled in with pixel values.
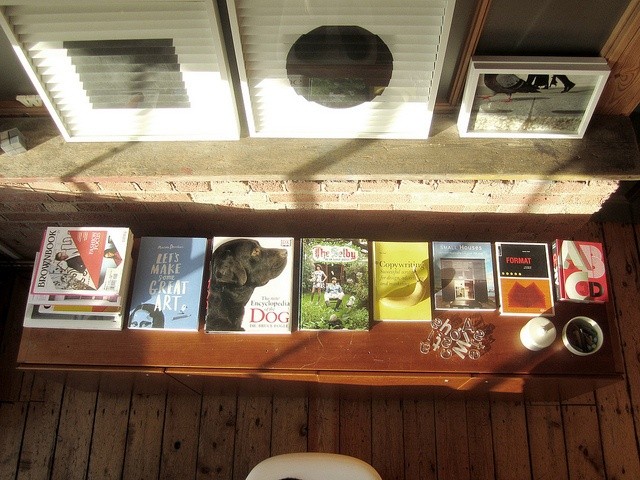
left=494, top=243, right=556, bottom=317
left=127, top=236, right=208, bottom=332
left=204, top=236, right=295, bottom=335
left=432, top=241, right=496, bottom=312
left=22, top=226, right=135, bottom=331
left=297, top=237, right=370, bottom=332
left=552, top=239, right=609, bottom=305
left=372, top=241, right=433, bottom=322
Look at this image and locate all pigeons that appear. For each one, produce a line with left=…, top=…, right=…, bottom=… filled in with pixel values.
left=478, top=74, right=540, bottom=102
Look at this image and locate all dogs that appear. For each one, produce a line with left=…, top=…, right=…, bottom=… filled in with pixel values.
left=205, top=239, right=286, bottom=332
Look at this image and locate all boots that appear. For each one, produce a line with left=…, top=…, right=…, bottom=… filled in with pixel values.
left=324, top=301, right=331, bottom=309
left=334, top=298, right=342, bottom=313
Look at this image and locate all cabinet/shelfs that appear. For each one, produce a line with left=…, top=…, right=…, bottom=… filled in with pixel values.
left=18, top=236, right=321, bottom=401
left=317, top=237, right=625, bottom=404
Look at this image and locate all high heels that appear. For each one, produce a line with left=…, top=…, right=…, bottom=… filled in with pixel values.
left=315, top=301, right=321, bottom=306
left=309, top=300, right=313, bottom=306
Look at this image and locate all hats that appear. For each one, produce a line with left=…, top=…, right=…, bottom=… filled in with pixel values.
left=315, top=263, right=322, bottom=269
left=331, top=276, right=337, bottom=280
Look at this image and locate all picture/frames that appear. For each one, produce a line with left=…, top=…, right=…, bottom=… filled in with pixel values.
left=458, top=56, right=612, bottom=143
